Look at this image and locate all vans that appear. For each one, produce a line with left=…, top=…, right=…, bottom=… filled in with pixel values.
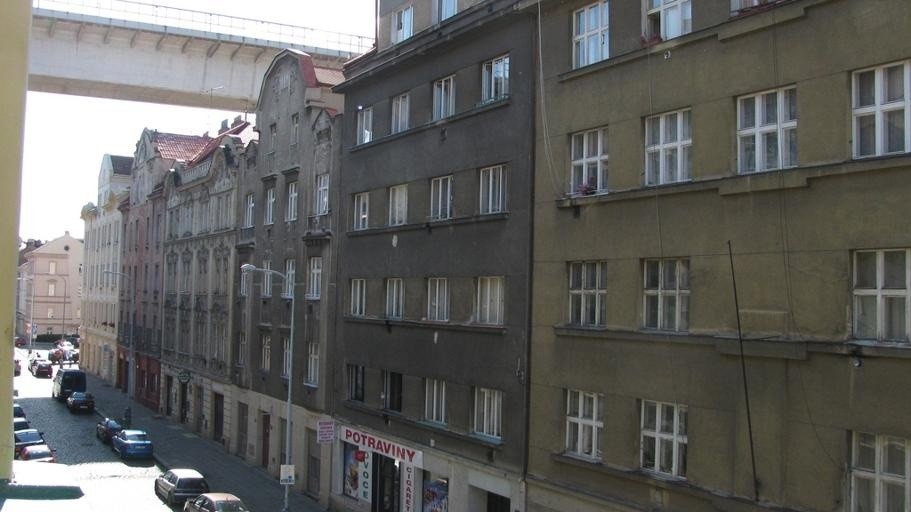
left=53, top=368, right=86, bottom=400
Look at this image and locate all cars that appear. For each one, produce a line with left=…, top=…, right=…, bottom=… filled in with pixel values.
left=13, top=335, right=26, bottom=349
left=96, top=416, right=154, bottom=459
left=182, top=492, right=250, bottom=512
left=14, top=398, right=55, bottom=463
left=67, top=390, right=95, bottom=413
left=28, top=356, right=53, bottom=377
left=155, top=469, right=209, bottom=506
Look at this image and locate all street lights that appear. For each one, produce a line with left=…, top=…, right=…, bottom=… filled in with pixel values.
left=102, top=263, right=135, bottom=420
left=239, top=263, right=295, bottom=512
left=16, top=274, right=36, bottom=354
left=46, top=269, right=67, bottom=362
